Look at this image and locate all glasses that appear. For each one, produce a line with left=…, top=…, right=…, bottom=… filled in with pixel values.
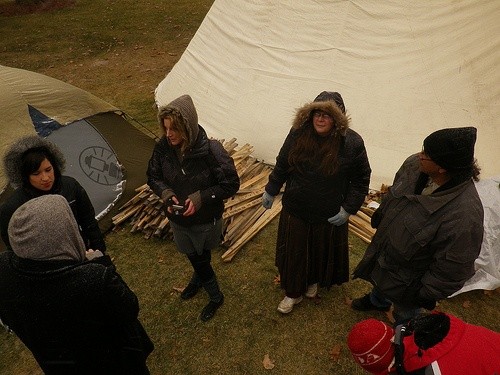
left=312, top=111, right=332, bottom=120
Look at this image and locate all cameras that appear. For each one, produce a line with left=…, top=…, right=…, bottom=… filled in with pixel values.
left=172, top=205, right=187, bottom=215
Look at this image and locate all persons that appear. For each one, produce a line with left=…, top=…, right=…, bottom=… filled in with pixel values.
left=146, top=94, right=240, bottom=321
left=0, top=194, right=156, bottom=375
left=351, top=126, right=484, bottom=328
left=0, top=135, right=107, bottom=259
left=262, top=90, right=372, bottom=313
left=345, top=310, right=500, bottom=375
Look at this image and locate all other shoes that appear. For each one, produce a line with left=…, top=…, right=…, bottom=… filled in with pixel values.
left=200, top=295, right=225, bottom=320
left=351, top=292, right=391, bottom=313
left=180, top=280, right=203, bottom=299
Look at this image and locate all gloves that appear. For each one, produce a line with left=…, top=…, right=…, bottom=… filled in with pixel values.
left=262, top=189, right=274, bottom=209
left=327, top=207, right=350, bottom=226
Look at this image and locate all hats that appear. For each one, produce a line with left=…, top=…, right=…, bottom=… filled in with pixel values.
left=424, top=127, right=477, bottom=173
left=346, top=319, right=397, bottom=375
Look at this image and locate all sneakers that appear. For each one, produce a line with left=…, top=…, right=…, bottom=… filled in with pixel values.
left=305, top=282, right=318, bottom=298
left=278, top=294, right=304, bottom=313
